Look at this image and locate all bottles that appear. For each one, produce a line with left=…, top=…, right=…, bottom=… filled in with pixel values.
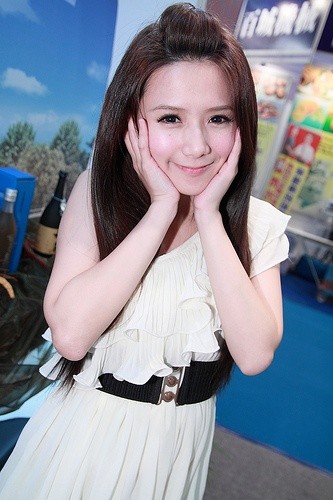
left=31, top=170, right=72, bottom=257
left=0, top=187, right=17, bottom=280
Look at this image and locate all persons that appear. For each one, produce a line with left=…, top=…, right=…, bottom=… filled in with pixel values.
left=0, top=2, right=291, bottom=498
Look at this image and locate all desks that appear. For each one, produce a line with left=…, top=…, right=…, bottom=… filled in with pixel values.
left=285, top=225, right=333, bottom=303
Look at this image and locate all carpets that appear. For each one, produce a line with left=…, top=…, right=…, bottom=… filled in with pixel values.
left=216, top=274, right=333, bottom=480
